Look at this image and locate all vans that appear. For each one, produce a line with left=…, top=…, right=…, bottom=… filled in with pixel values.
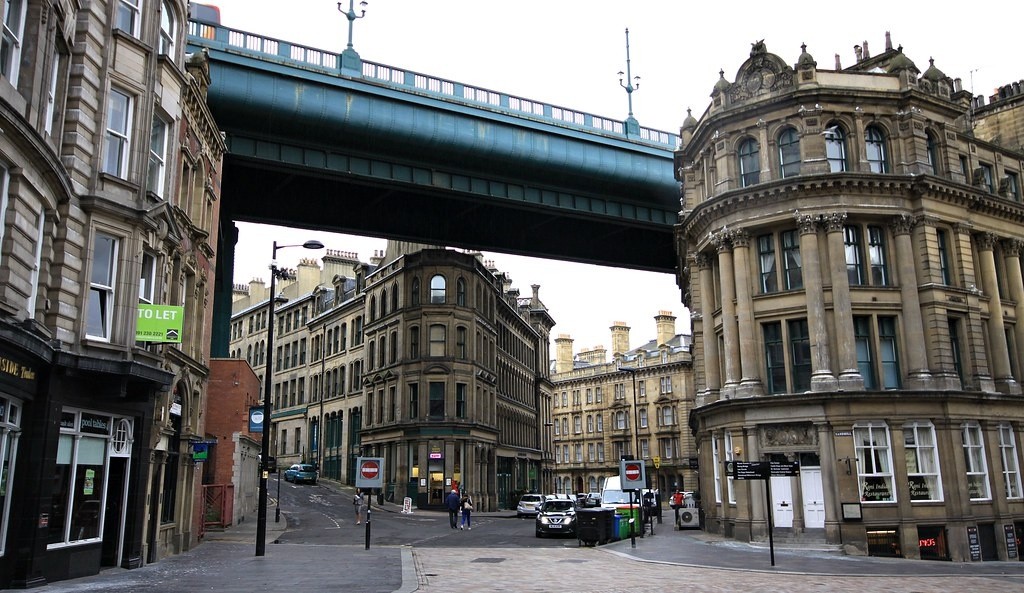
left=601, top=475, right=644, bottom=521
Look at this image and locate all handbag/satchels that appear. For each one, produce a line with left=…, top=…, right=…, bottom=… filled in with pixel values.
left=464, top=499, right=473, bottom=510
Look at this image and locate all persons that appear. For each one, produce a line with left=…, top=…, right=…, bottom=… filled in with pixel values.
left=445, top=489, right=460, bottom=529
left=673, top=490, right=685, bottom=507
left=459, top=493, right=473, bottom=531
left=354, top=488, right=365, bottom=525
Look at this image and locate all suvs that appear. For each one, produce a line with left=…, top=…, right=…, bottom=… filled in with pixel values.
left=640, top=489, right=658, bottom=507
left=669, top=491, right=695, bottom=509
left=257, top=454, right=277, bottom=473
left=534, top=499, right=580, bottom=539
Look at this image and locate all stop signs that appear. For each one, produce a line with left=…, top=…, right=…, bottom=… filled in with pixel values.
left=361, top=461, right=379, bottom=480
left=625, top=464, right=640, bottom=482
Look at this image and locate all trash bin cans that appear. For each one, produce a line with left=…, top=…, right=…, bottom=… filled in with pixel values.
left=616, top=506, right=641, bottom=536
left=378, top=493, right=384, bottom=505
left=574, top=506, right=630, bottom=547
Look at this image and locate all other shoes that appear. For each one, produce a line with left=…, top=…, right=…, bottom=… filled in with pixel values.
left=357, top=521, right=360, bottom=525
left=468, top=527, right=472, bottom=530
left=461, top=526, right=463, bottom=530
left=454, top=523, right=457, bottom=529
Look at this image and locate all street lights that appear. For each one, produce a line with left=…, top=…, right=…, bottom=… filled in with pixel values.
left=617, top=26, right=640, bottom=139
left=337, top=0, right=368, bottom=78
left=619, top=366, right=639, bottom=460
left=255, top=238, right=324, bottom=558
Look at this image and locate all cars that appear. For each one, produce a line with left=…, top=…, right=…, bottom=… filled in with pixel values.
left=545, top=492, right=602, bottom=508
left=517, top=494, right=544, bottom=518
left=284, top=464, right=319, bottom=486
